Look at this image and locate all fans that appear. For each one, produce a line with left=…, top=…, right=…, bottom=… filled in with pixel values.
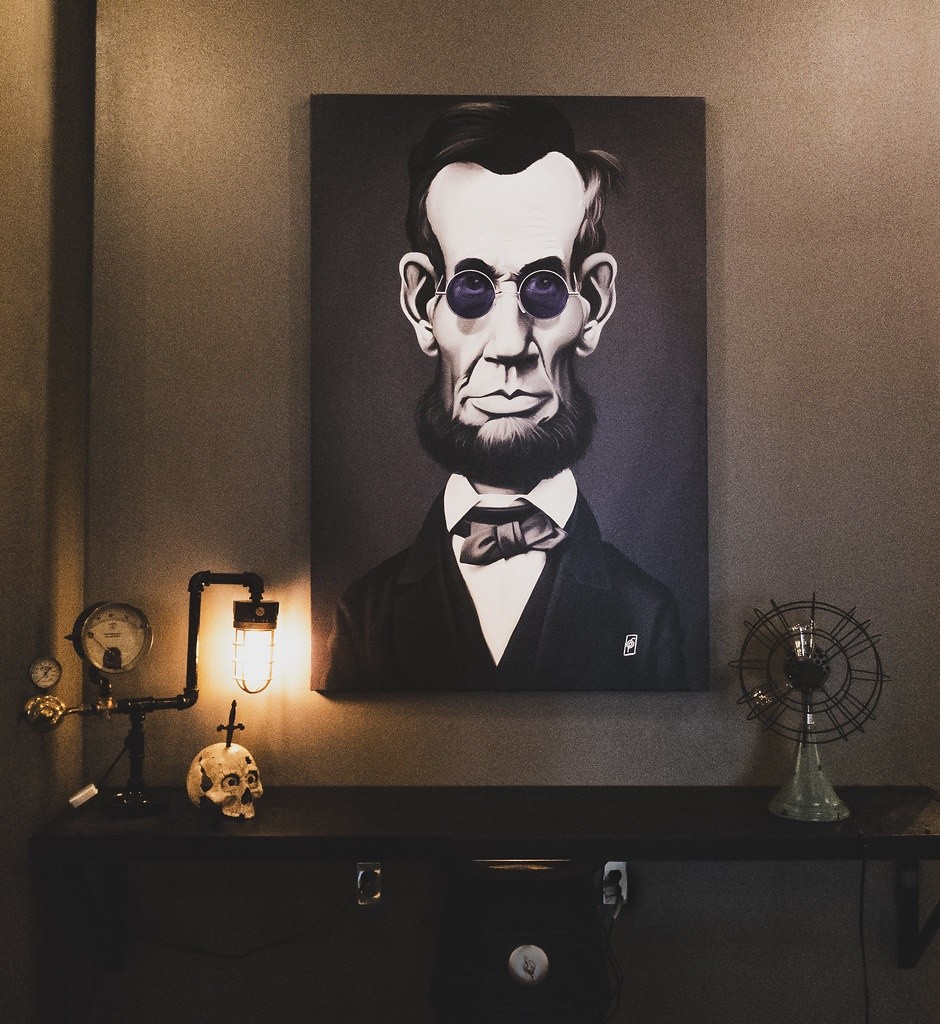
left=726, top=592, right=893, bottom=825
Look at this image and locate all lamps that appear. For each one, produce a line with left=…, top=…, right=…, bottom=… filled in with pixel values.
left=20, top=571, right=281, bottom=816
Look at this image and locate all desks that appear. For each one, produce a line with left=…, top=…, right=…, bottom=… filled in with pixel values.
left=36, top=784, right=940, bottom=1024
